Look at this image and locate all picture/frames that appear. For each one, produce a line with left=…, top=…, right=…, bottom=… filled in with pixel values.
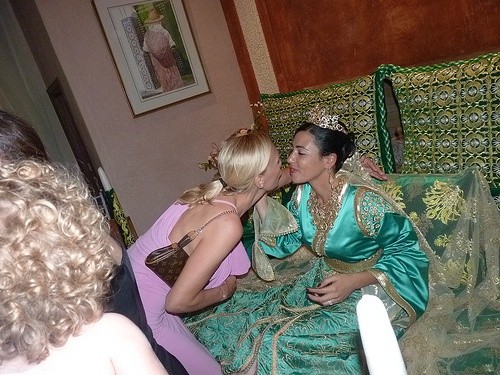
left=93, top=0, right=214, bottom=120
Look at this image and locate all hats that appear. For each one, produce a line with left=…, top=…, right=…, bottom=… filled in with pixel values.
left=143, top=9, right=165, bottom=24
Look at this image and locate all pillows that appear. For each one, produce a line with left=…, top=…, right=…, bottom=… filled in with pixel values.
left=384, top=52, right=500, bottom=199
left=242, top=181, right=299, bottom=236
left=260, top=71, right=397, bottom=177
left=375, top=169, right=485, bottom=295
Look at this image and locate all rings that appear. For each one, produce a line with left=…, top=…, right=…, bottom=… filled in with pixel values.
left=327, top=300, right=333, bottom=306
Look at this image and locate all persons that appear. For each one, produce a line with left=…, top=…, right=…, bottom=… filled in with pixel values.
left=103, top=129, right=389, bottom=375
left=1, top=156, right=170, bottom=375
left=0, top=109, right=191, bottom=375
left=176, top=102, right=431, bottom=375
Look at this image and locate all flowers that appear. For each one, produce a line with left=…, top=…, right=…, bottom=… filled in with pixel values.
left=196, top=123, right=255, bottom=176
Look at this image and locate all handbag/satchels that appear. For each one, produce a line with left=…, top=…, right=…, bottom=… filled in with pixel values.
left=145, top=30, right=174, bottom=68
left=143, top=208, right=243, bottom=301
